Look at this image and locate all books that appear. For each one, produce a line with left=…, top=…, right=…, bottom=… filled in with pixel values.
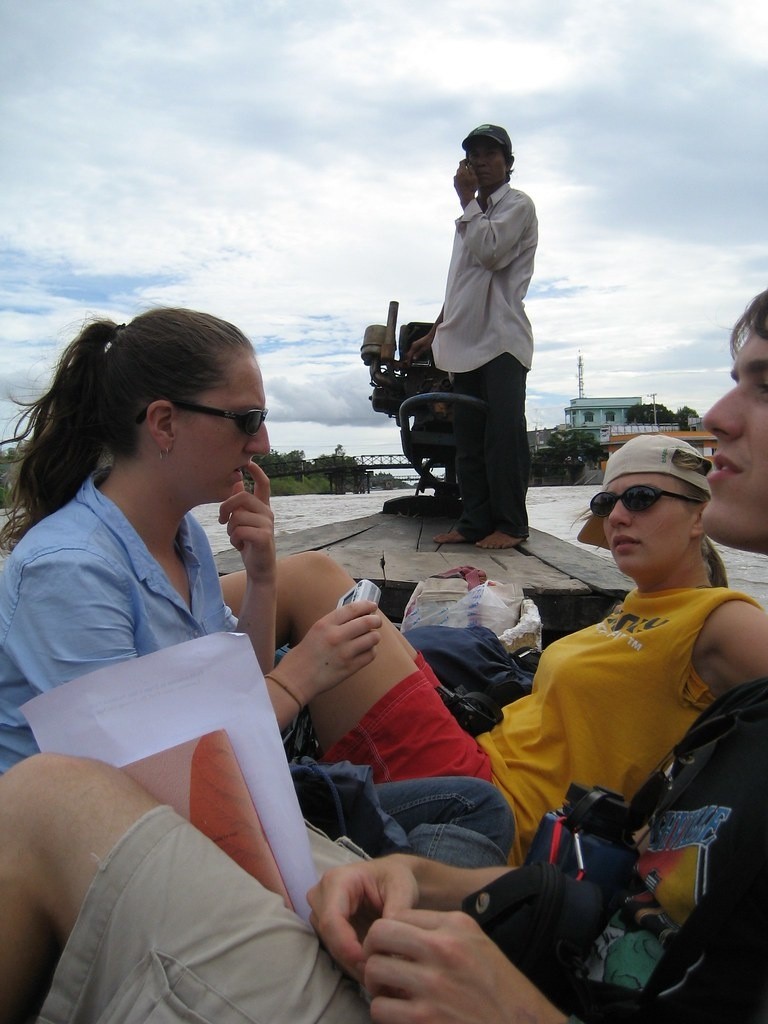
left=122, top=729, right=295, bottom=913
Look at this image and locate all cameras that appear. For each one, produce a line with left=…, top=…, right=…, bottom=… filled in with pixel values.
left=336, top=580, right=382, bottom=616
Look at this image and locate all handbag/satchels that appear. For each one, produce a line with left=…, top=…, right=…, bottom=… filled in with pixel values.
left=401, top=567, right=524, bottom=653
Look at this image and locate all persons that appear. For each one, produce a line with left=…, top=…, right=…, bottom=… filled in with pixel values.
left=1, top=289, right=768, bottom=1024
left=406, top=124, right=538, bottom=549
left=219, top=436, right=768, bottom=867
left=0, top=307, right=515, bottom=867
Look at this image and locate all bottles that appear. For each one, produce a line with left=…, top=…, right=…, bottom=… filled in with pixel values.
left=526, top=781, right=638, bottom=911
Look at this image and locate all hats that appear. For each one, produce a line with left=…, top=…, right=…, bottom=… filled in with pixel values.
left=578, top=434, right=714, bottom=550
left=462, top=125, right=511, bottom=155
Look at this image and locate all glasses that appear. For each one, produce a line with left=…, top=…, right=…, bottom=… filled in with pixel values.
left=590, top=484, right=704, bottom=517
left=135, top=397, right=268, bottom=437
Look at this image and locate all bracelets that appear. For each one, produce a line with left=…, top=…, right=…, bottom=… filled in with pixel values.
left=265, top=675, right=303, bottom=709
